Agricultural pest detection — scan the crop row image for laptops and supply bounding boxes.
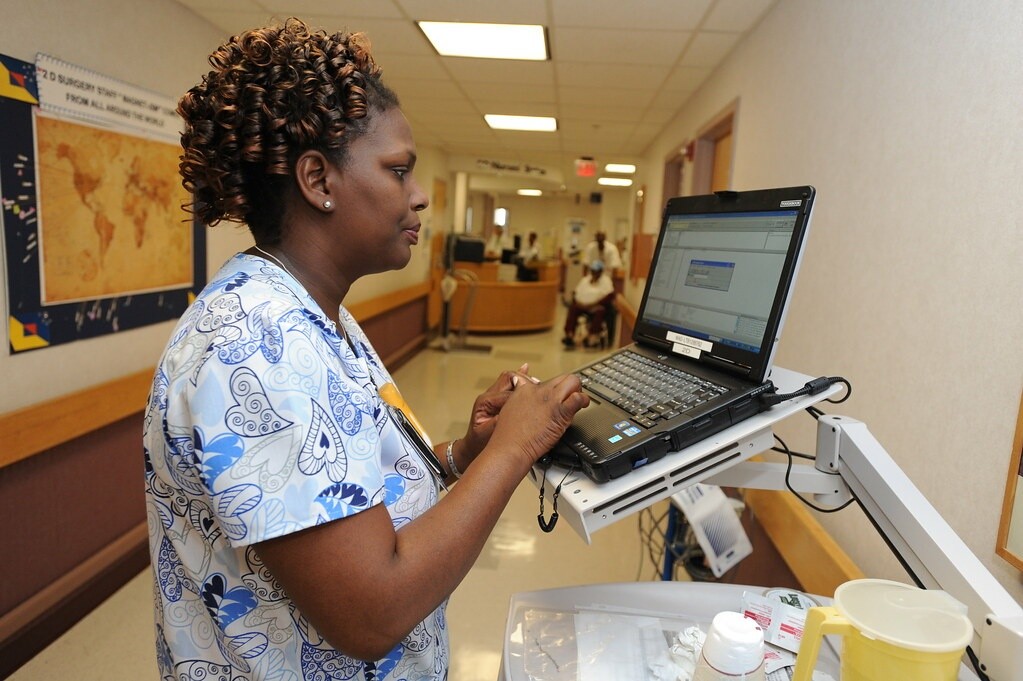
[544,185,816,484]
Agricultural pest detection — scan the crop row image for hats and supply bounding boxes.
[590,260,603,270]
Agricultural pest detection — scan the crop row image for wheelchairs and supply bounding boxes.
[561,294,618,354]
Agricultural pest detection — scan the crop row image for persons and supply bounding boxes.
[484,221,544,283]
[560,260,614,347]
[138,22,594,681]
[580,228,623,347]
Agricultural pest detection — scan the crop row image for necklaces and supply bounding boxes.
[252,245,349,342]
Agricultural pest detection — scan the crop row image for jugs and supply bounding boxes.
[791,578,972,681]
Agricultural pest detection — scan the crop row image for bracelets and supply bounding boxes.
[447,438,467,478]
